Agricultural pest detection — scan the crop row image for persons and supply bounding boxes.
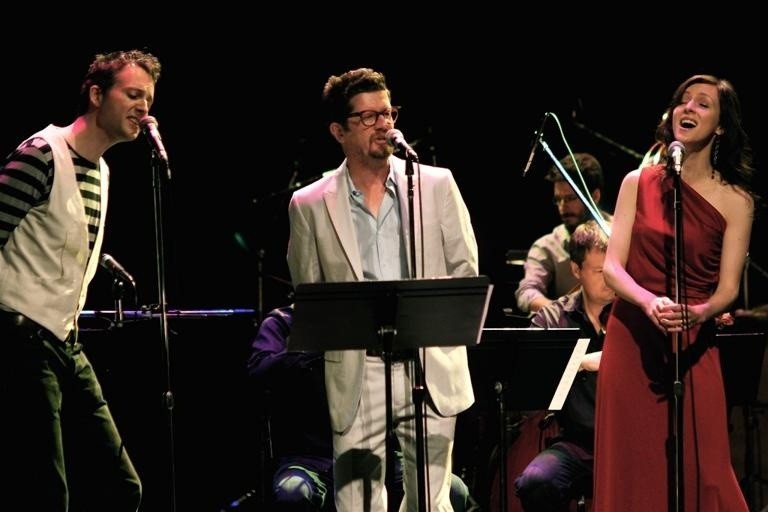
[595,72,761,510]
[240,298,340,510]
[286,66,484,510]
[0,48,162,512]
[512,151,625,333]
[511,220,622,511]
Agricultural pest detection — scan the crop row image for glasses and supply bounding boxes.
[340,105,400,129]
[552,195,580,206]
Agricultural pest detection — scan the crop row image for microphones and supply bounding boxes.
[98,253,134,283]
[427,126,437,166]
[139,116,169,169]
[521,113,550,179]
[385,128,419,161]
[668,141,685,177]
